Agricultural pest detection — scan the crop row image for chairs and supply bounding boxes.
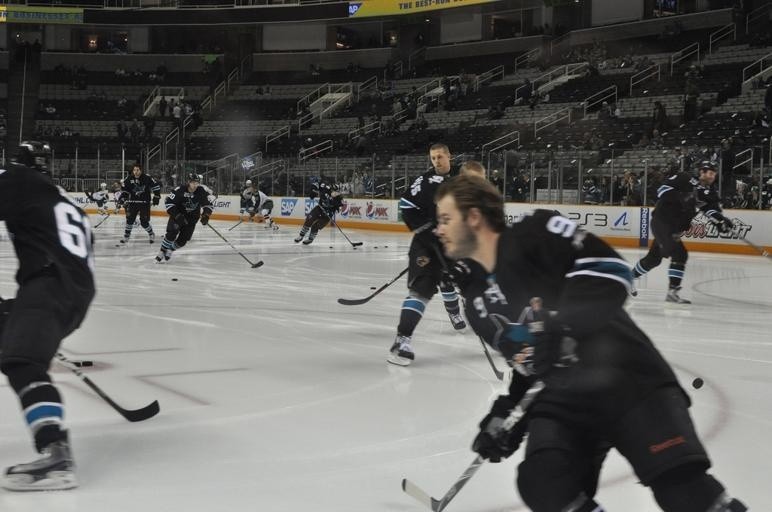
[1,18,771,213]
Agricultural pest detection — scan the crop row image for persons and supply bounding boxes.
[0,164,97,478]
[387,160,487,359]
[432,172,749,511]
[426,143,467,329]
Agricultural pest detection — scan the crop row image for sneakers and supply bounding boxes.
[665,287,691,304]
[155,250,165,262]
[116,239,128,246]
[302,238,313,244]
[391,325,415,361]
[6,440,74,474]
[294,235,303,242]
[448,313,467,330]
[149,232,155,244]
[165,248,173,260]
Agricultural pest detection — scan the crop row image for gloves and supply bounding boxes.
[710,213,733,233]
[471,395,528,463]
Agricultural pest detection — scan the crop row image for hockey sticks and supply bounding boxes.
[399,379,547,512]
[197,213,264,269]
[338,267,408,305]
[316,200,362,247]
[52,350,159,422]
[84,189,146,204]
[431,246,510,382]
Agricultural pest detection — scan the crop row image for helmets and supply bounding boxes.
[329,183,340,196]
[186,172,200,188]
[698,160,719,172]
[10,140,53,179]
